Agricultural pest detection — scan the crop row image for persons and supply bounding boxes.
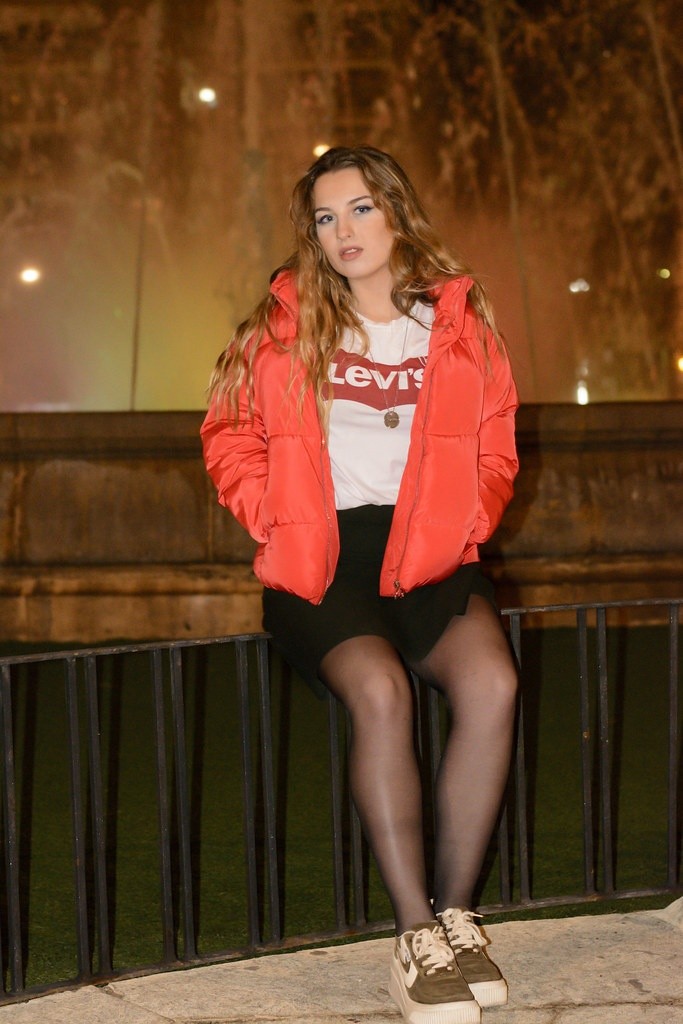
[196,144,523,1024]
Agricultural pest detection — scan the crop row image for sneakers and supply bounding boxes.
[435,905,509,1009]
[388,919,481,1023]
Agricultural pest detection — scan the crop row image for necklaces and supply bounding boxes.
[341,285,419,428]
